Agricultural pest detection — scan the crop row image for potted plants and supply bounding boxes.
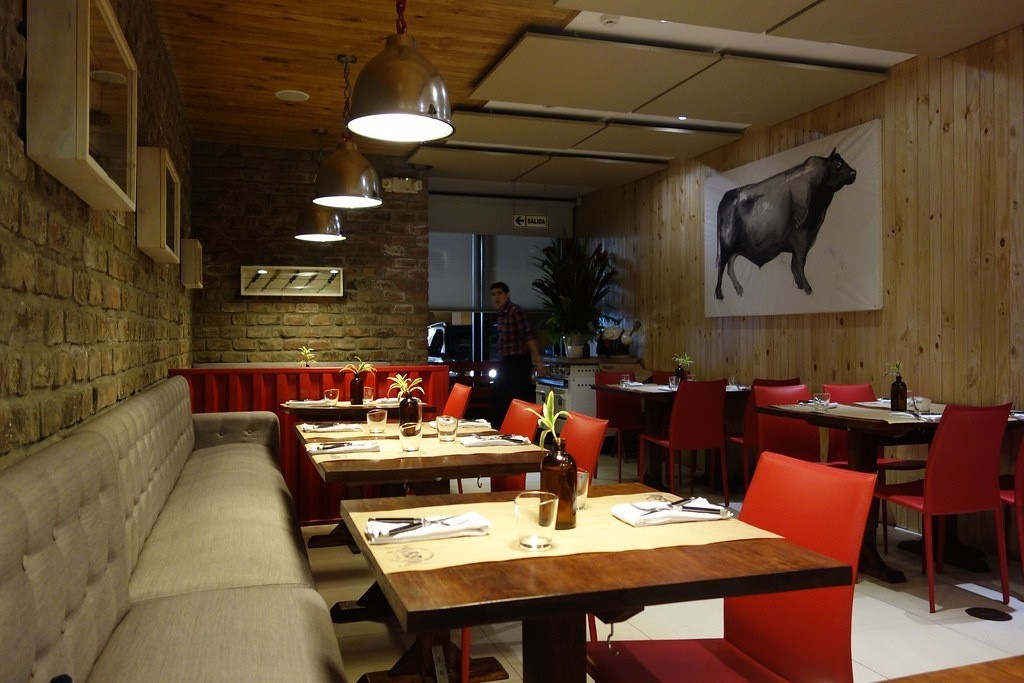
[526,222,618,357]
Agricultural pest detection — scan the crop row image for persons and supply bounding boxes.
[485,282,547,421]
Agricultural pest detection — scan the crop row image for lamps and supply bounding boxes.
[292,0,455,244]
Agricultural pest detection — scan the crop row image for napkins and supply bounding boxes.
[877,397,914,405]
[375,397,421,403]
[458,434,532,446]
[301,423,363,433]
[430,419,491,429]
[611,497,734,527]
[800,399,838,409]
[889,412,943,421]
[658,386,678,391]
[364,509,490,545]
[620,381,643,386]
[286,399,326,405]
[306,440,381,454]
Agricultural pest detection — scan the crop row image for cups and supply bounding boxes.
[812,393,831,414]
[912,393,932,416]
[436,414,458,442]
[513,489,560,550]
[573,466,589,510]
[323,388,339,407]
[366,409,388,432]
[364,386,373,403]
[618,374,630,387]
[687,374,697,381]
[668,375,680,388]
[399,422,421,452]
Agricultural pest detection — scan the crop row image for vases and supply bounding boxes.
[890,376,907,411]
[349,372,363,404]
[398,392,423,431]
[674,364,684,381]
[538,438,577,530]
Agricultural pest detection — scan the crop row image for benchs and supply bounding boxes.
[63,374,317,602]
[2,429,347,683]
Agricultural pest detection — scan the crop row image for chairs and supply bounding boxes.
[427,367,1024,683]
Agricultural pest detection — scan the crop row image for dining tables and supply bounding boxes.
[291,417,551,554]
[338,479,853,683]
[277,395,437,549]
[589,379,753,492]
[754,396,1024,585]
